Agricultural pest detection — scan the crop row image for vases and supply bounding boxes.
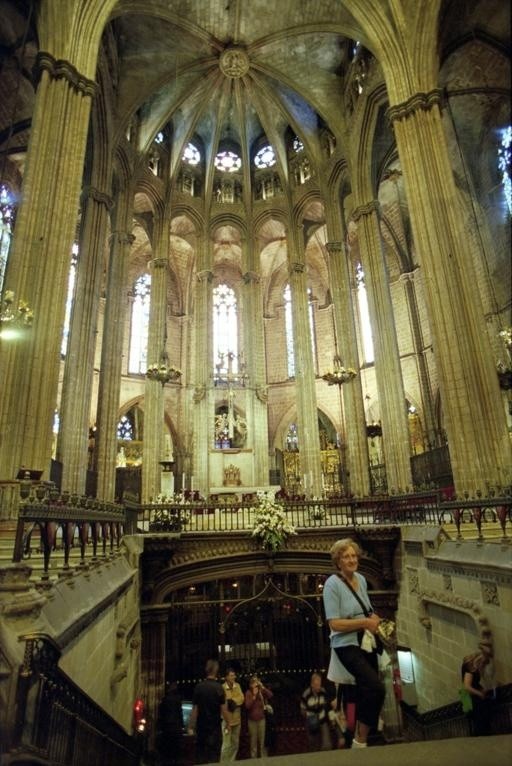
[149,521,181,532]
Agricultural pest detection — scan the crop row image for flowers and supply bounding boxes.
[307,507,327,526]
[146,364,182,382]
[322,368,357,383]
[252,491,298,551]
[153,494,190,523]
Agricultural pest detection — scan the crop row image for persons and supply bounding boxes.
[459,649,493,738]
[322,536,387,748]
[158,659,387,766]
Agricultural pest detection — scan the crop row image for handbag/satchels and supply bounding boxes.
[263,704,274,716]
[308,714,320,732]
[357,629,384,655]
